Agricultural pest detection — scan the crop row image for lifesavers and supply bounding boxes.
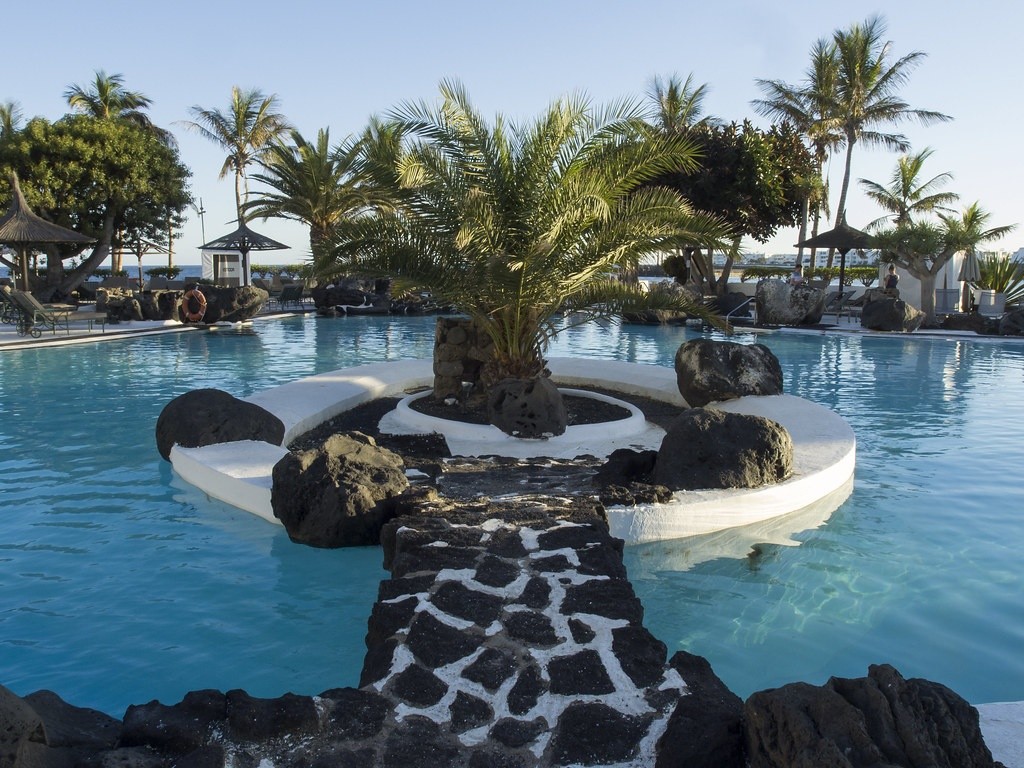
[182,289,207,321]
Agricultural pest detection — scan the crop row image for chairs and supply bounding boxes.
[823,288,864,326]
[250,274,314,309]
[9,289,106,336]
[0,284,77,336]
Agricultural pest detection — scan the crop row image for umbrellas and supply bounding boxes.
[960,237,981,315]
[790,207,895,310]
[0,204,100,338]
[195,218,291,286]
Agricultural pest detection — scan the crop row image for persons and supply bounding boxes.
[790,264,803,285]
[884,264,900,289]
